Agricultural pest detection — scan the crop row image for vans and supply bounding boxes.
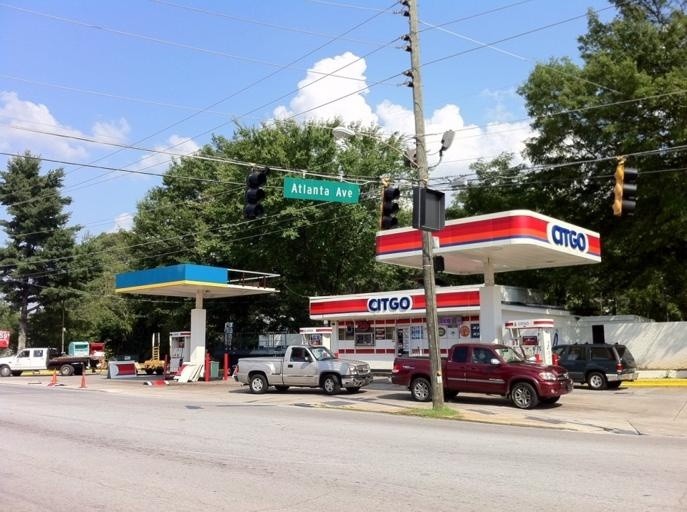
[550,342,637,388]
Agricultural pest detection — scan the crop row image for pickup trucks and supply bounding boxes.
[234,344,373,393]
[390,343,573,409]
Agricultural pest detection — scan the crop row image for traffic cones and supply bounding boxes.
[48,372,56,387]
[80,371,86,389]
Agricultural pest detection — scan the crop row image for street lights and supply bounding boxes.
[331,128,454,408]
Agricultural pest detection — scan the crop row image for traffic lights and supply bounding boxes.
[612,166,641,217]
[379,185,401,234]
[244,171,266,220]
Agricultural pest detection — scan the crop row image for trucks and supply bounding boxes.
[0,348,104,377]
